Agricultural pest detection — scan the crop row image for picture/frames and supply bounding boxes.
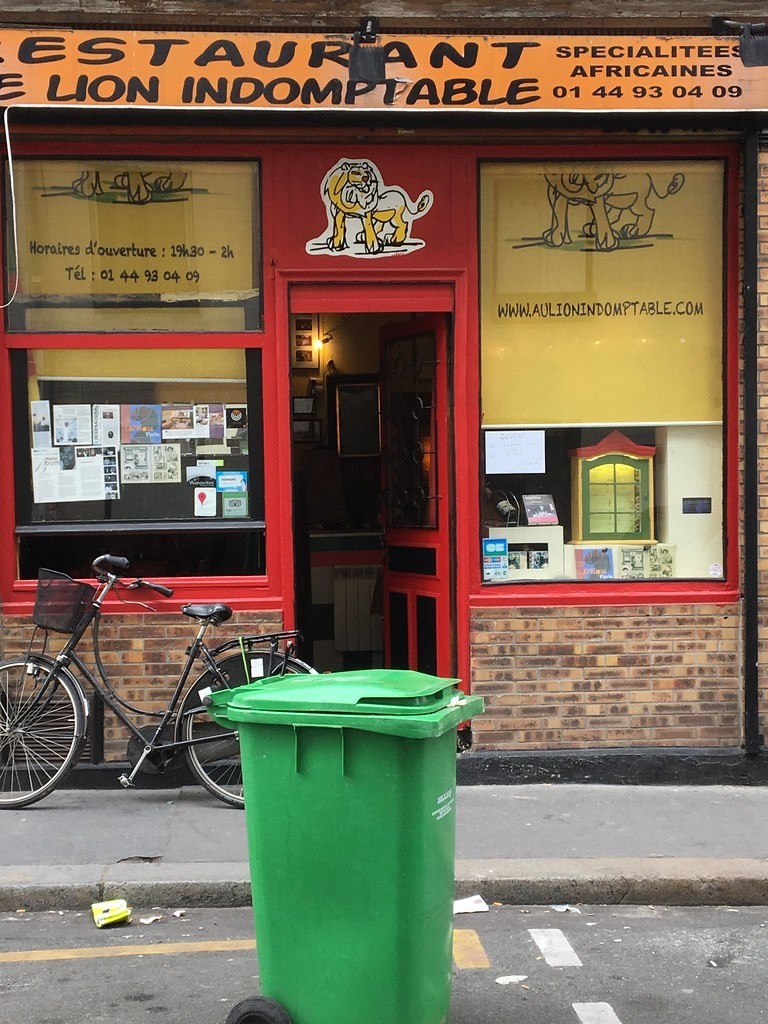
[288,313,320,371]
[293,418,324,449]
[292,396,317,415]
[325,372,389,466]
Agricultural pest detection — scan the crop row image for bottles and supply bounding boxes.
[484,487,517,522]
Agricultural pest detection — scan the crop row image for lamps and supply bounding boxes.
[711,16,768,67]
[348,15,386,85]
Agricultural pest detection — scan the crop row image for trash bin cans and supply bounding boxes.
[203,667,485,1024]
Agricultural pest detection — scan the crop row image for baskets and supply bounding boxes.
[33,567,97,634]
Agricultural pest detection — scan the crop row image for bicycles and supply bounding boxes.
[0,552,321,810]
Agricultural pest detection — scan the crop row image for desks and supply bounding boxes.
[308,523,386,675]
